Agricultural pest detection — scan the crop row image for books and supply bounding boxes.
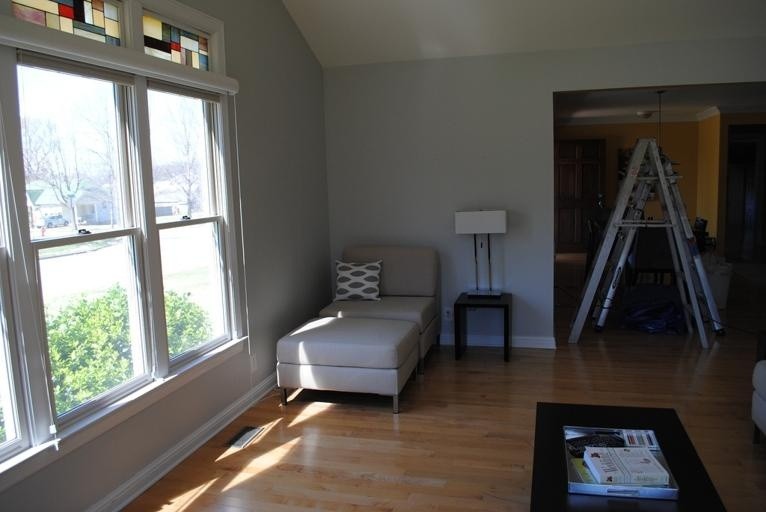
[583,445,670,487]
[563,426,680,500]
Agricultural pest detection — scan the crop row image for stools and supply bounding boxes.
[275,316,419,416]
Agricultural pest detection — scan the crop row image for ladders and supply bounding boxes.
[568,137,710,352]
[593,148,728,338]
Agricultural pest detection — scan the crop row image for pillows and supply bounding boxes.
[331,258,382,301]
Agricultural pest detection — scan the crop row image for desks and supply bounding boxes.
[454,292,513,362]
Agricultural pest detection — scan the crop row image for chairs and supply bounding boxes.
[319,246,441,374]
[586,218,668,296]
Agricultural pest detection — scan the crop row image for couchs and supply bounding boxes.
[748,331,765,444]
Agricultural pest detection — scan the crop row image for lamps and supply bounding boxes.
[636,112,652,118]
[454,209,507,298]
